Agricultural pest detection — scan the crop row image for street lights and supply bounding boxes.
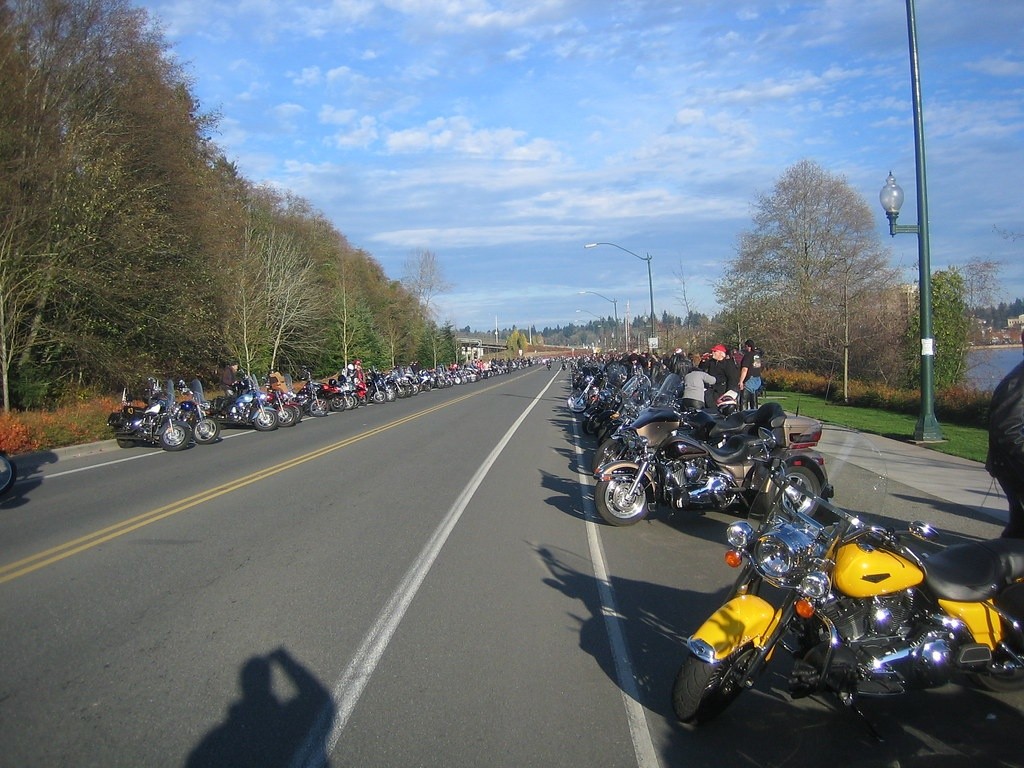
[583,292,618,350]
[880,173,944,441]
[584,243,654,354]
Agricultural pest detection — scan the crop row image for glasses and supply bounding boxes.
[711,350,717,352]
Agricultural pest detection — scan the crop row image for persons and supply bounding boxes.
[985,329,1024,541]
[300,365,311,381]
[347,359,361,383]
[408,356,542,380]
[547,338,765,412]
[221,362,239,396]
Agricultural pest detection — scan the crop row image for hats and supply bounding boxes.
[673,348,682,354]
[231,361,238,366]
[710,344,727,352]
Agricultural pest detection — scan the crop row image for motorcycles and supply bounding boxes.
[0,451,19,493]
[672,430,1024,745]
[108,380,221,452]
[204,353,542,426]
[567,358,836,520]
[547,361,566,370]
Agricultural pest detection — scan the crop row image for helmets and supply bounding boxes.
[179,401,192,411]
[715,395,738,407]
[328,378,337,385]
